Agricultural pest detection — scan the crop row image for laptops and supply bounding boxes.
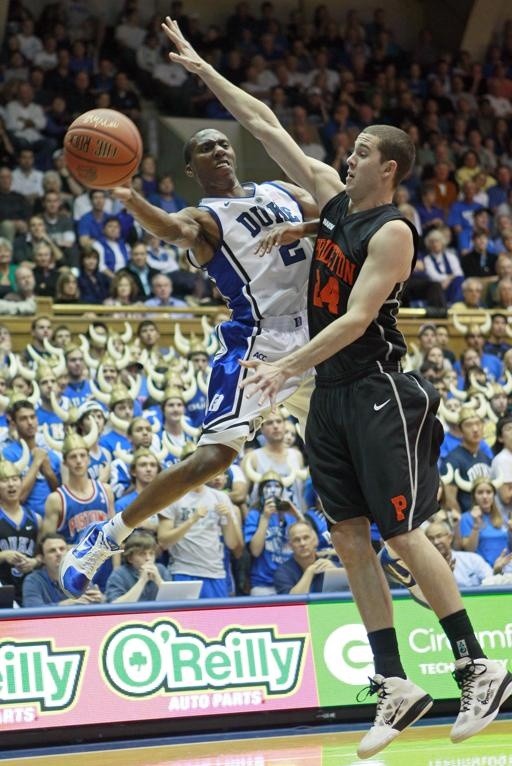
[155,581,203,600]
[322,568,350,592]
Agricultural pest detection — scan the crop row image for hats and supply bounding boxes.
[0,317,218,487]
[409,308,512,492]
[243,456,298,496]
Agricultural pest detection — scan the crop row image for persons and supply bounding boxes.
[60,126,431,609]
[159,12,512,759]
[169,1,512,317]
[0,311,342,608]
[2,3,229,303]
[400,311,512,585]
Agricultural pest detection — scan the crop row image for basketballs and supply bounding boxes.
[65,109,143,186]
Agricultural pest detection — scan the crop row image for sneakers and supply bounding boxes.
[355,672,435,761]
[57,519,122,601]
[376,543,434,611]
[448,654,512,744]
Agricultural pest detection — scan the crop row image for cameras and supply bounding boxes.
[275,498,290,512]
